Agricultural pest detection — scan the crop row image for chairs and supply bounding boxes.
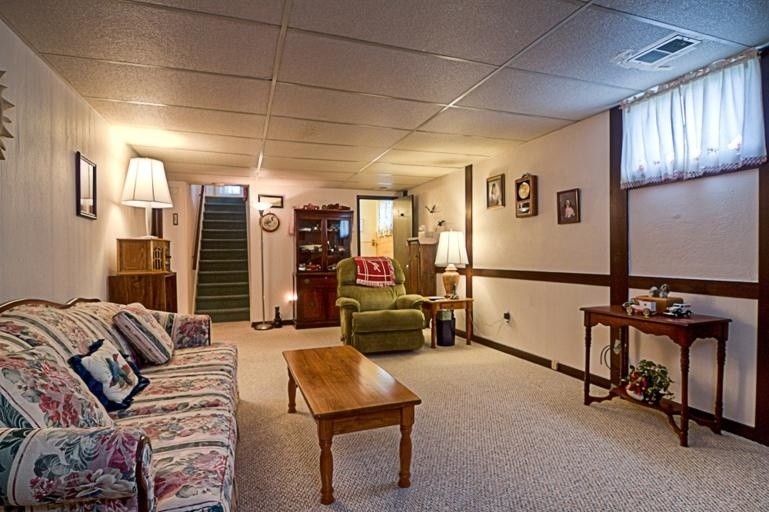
[335,256,429,354]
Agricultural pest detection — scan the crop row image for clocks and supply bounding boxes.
[515,173,538,218]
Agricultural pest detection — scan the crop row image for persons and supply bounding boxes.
[565,199,576,219]
[490,183,500,201]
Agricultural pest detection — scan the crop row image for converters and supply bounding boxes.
[504,313,509,319]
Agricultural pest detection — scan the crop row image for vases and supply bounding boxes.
[625,385,665,405]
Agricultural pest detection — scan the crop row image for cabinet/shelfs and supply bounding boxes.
[108,239,177,313]
[293,209,354,329]
[406,244,437,328]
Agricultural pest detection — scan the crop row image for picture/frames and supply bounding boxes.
[76,151,97,219]
[173,213,178,225]
[258,194,283,208]
[487,173,505,208]
[556,188,580,224]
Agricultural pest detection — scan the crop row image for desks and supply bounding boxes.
[422,296,474,349]
[579,305,733,447]
[282,344,421,505]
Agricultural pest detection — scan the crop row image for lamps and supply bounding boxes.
[434,228,470,298]
[255,202,273,330]
[121,157,174,239]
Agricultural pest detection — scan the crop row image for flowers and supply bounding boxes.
[620,360,676,404]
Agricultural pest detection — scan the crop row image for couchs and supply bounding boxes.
[0,297,240,512]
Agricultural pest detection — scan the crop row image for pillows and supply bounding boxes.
[68,338,150,412]
[0,343,116,429]
[112,301,174,365]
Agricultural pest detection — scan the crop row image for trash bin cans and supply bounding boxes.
[436,316,455,346]
